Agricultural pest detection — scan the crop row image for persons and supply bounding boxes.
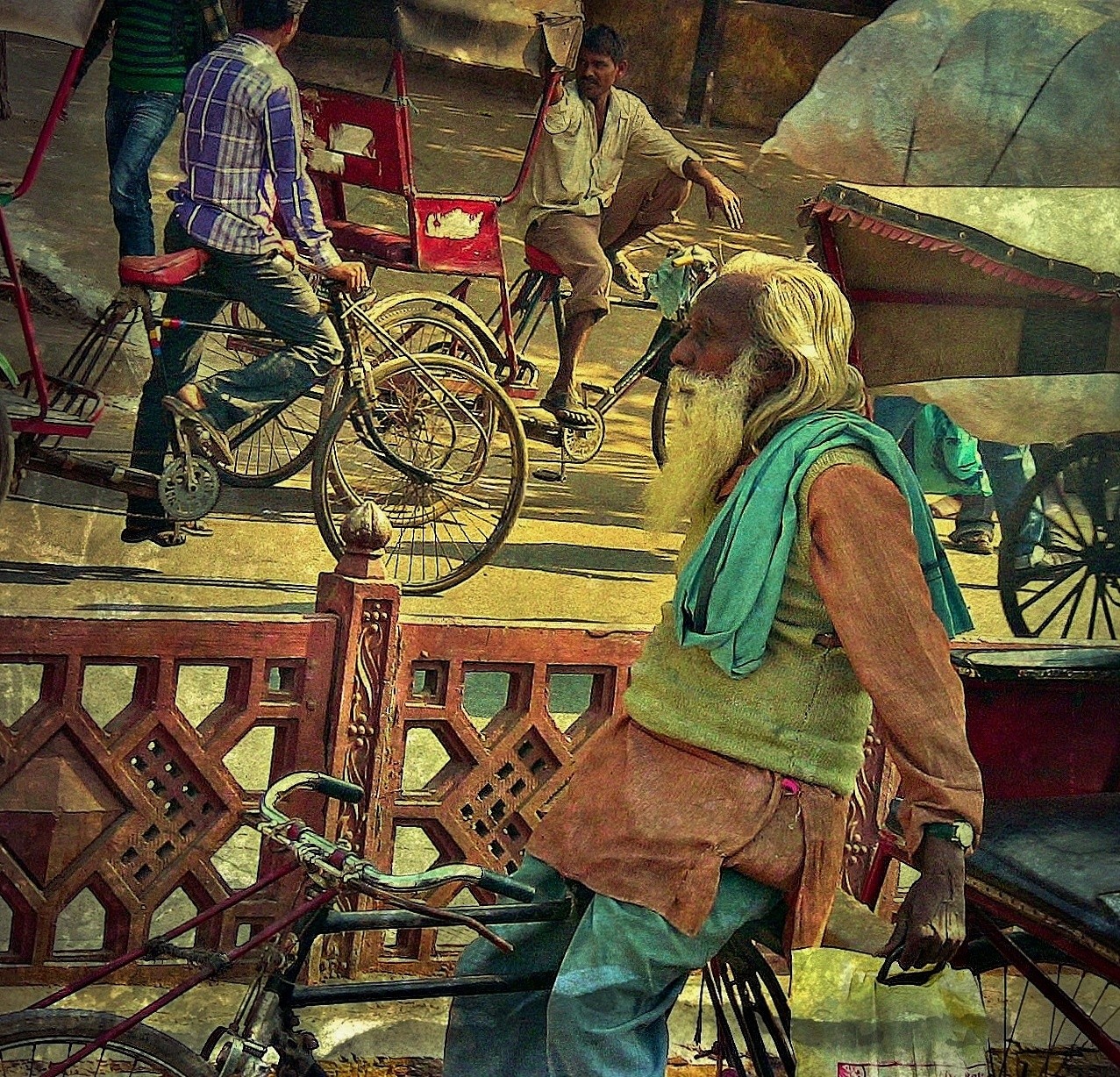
[514,21,744,429]
[118,0,370,549]
[439,244,986,1077]
[930,430,1081,572]
[71,0,231,289]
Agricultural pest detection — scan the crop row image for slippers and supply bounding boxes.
[603,249,643,293]
[539,397,596,431]
[120,517,214,544]
[162,394,235,464]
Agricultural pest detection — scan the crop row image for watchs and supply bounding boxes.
[923,817,975,851]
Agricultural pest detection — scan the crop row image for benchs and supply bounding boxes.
[295,78,521,386]
[859,639,1120,982]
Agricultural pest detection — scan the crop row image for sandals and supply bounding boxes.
[959,533,995,556]
[1040,527,1074,570]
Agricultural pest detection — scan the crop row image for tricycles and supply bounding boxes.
[0,0,528,596]
[181,0,721,487]
[0,187,1120,1076]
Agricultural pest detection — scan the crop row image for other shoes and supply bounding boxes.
[108,291,164,324]
